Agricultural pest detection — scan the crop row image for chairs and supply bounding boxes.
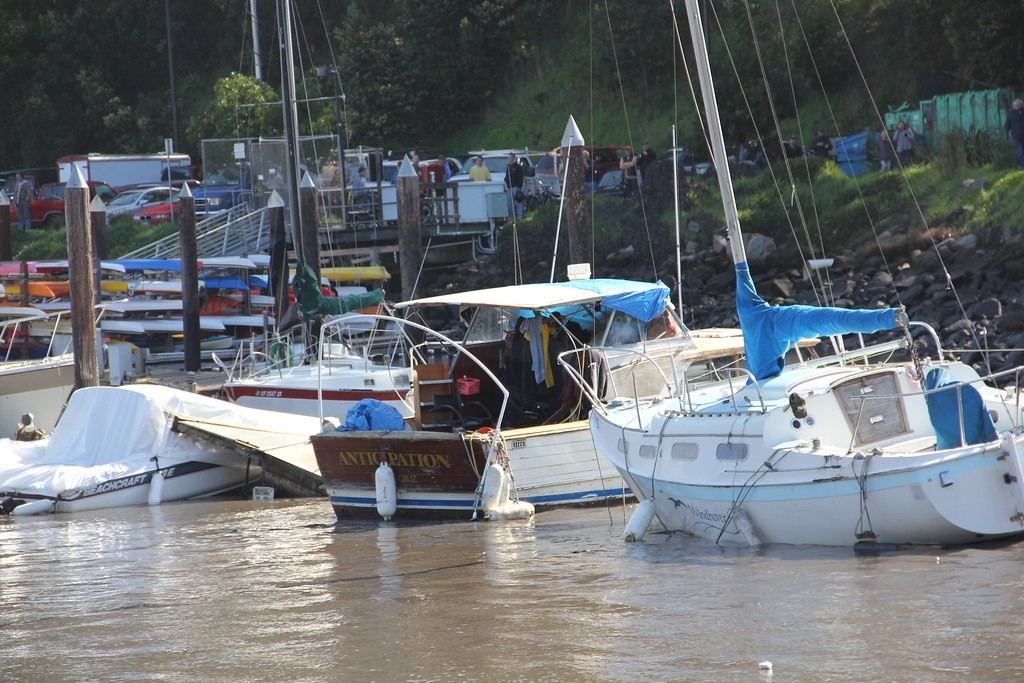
[432,392,493,431]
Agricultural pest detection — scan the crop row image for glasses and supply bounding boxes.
[336,173,340,176]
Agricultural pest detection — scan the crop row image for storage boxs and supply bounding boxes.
[458,378,480,395]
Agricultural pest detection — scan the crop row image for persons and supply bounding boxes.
[504,153,523,219]
[583,151,596,194]
[620,146,637,196]
[637,142,656,183]
[391,161,402,184]
[893,121,914,168]
[812,129,833,157]
[412,154,450,196]
[780,135,803,158]
[469,156,491,181]
[1005,99,1024,167]
[13,173,33,231]
[346,167,379,227]
[877,131,892,172]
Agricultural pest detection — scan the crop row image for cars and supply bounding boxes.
[7,180,117,233]
[300,161,328,205]
[4,174,39,198]
[105,186,180,226]
[378,135,810,197]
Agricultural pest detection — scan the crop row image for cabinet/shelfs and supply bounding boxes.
[413,362,453,430]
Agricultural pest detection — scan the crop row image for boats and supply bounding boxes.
[0,257,391,366]
[0,383,340,515]
[310,258,823,522]
[224,339,415,420]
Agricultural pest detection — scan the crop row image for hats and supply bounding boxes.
[21,413,34,421]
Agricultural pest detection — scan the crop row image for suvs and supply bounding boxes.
[190,162,279,221]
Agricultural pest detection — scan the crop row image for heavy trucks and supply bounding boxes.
[55,148,202,187]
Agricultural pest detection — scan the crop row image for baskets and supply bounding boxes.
[457,377,480,396]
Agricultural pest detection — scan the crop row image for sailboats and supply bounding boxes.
[558,0,1022,552]
